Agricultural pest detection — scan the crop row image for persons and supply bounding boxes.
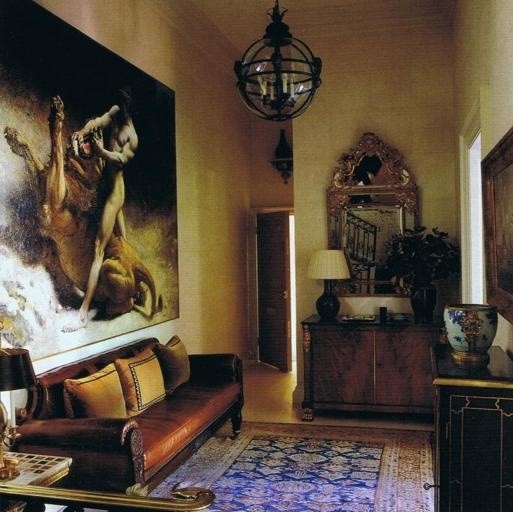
[61,85,139,332]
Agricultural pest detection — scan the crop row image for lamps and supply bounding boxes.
[307,250,351,319]
[0,349,37,482]
[268,129,294,184]
[234,1,323,122]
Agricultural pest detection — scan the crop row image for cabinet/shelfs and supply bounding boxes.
[431,345,512,512]
[300,314,448,421]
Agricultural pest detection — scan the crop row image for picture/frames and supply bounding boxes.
[480,126,513,327]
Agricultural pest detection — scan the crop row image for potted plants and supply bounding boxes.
[386,225,459,321]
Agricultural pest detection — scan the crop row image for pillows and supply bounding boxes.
[114,346,166,418]
[155,335,191,393]
[63,363,127,423]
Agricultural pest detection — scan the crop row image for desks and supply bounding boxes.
[0,451,73,511]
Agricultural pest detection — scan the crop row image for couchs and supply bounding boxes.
[9,339,243,498]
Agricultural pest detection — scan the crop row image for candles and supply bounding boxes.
[10,391,15,427]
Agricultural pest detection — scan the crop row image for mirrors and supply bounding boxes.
[326,132,421,298]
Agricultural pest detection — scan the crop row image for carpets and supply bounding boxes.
[67,418,435,512]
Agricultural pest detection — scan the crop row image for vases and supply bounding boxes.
[441,304,498,363]
